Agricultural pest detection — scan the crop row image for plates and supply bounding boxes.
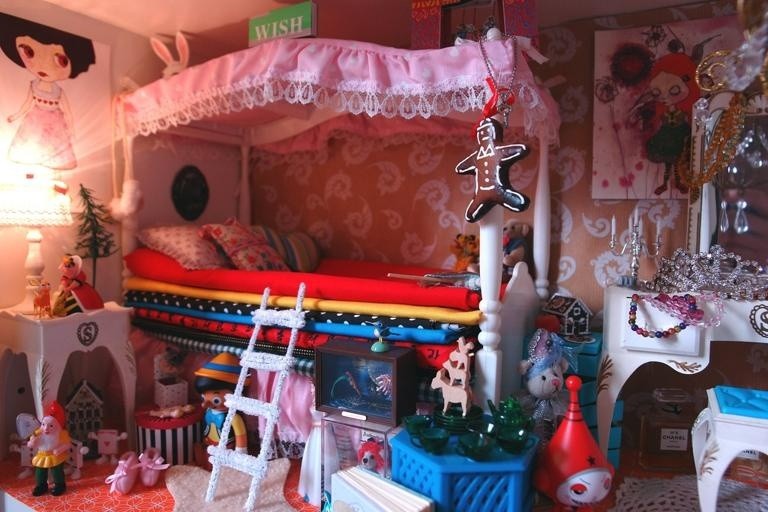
[433,402,485,435]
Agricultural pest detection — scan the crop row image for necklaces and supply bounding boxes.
[679,90,746,190]
[628,290,696,339]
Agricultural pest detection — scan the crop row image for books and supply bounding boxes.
[331,463,436,512]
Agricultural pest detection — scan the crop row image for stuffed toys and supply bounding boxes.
[518,327,574,424]
[356,438,391,478]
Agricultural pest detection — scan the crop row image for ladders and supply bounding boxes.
[203,283,311,512]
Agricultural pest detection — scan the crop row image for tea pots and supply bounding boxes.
[487,394,536,436]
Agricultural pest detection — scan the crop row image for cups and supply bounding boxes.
[457,427,492,463]
[491,428,537,457]
[408,428,449,456]
[400,414,433,436]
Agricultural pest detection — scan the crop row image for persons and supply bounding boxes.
[27,399,74,496]
[192,350,248,473]
[52,252,105,318]
